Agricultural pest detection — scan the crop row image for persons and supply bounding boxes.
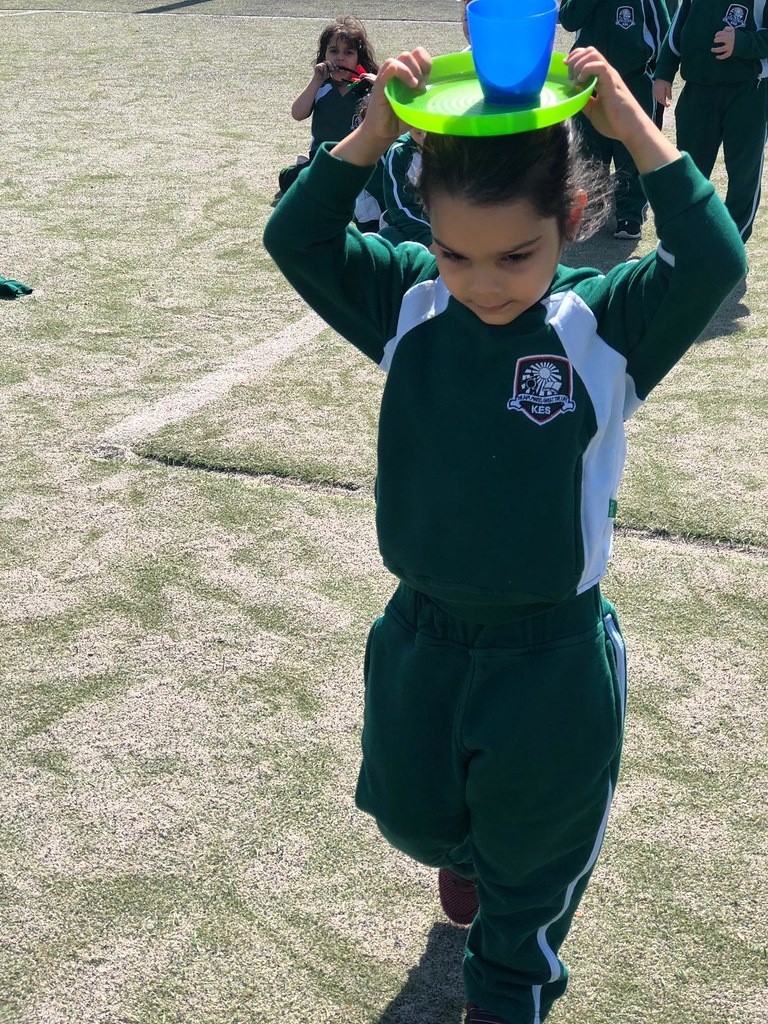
[460,0,471,52]
[559,0,671,240]
[651,0,768,242]
[279,15,432,247]
[263,45,749,1024]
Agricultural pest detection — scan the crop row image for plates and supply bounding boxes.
[384,52,598,137]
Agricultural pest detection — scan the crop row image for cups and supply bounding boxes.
[466,0,559,108]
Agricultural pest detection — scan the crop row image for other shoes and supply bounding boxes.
[438,867,479,924]
[465,1003,508,1024]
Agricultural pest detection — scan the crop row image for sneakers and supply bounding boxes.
[614,220,641,239]
